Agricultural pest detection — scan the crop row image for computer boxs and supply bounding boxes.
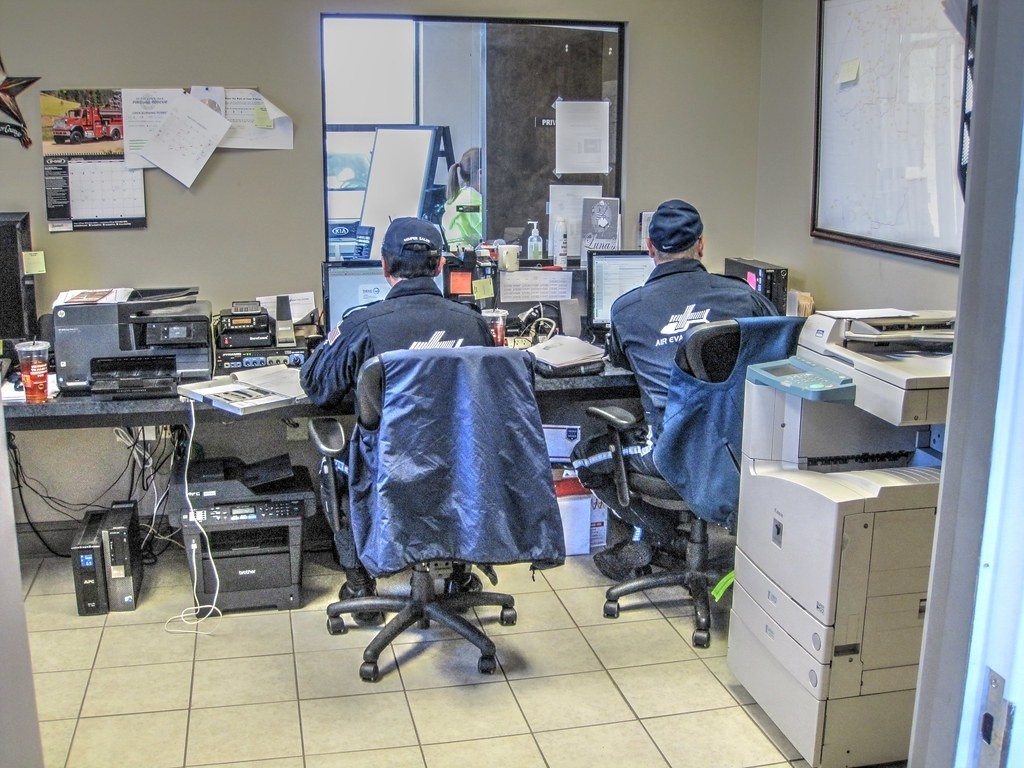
[724,258,788,315]
[0,212,39,368]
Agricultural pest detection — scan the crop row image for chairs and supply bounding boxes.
[326,346,567,682]
[388,214,451,253]
[586,316,808,648]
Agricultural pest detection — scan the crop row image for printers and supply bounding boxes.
[727,310,955,768]
[53,285,212,390]
[168,452,318,620]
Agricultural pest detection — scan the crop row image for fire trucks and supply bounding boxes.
[51,92,123,146]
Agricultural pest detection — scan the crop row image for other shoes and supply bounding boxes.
[650,529,690,560]
[444,572,483,613]
[339,581,380,625]
[593,539,652,582]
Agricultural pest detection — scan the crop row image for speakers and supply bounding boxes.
[559,299,581,338]
[276,296,296,347]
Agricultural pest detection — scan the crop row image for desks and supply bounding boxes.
[3,361,641,431]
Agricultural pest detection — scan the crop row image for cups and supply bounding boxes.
[15,341,51,404]
[481,309,509,347]
[498,245,519,271]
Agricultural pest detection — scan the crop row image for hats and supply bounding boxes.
[648,199,703,253]
[381,217,442,257]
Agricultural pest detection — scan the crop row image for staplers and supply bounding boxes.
[475,249,492,266]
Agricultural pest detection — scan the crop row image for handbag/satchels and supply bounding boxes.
[526,334,605,378]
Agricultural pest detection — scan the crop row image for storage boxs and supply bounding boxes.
[543,425,608,556]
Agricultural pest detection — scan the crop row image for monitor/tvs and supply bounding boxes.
[587,250,657,331]
[321,257,450,336]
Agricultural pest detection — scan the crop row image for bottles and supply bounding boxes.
[554,217,568,270]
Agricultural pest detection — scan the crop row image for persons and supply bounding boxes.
[441,147,484,254]
[300,217,495,628]
[570,200,781,582]
